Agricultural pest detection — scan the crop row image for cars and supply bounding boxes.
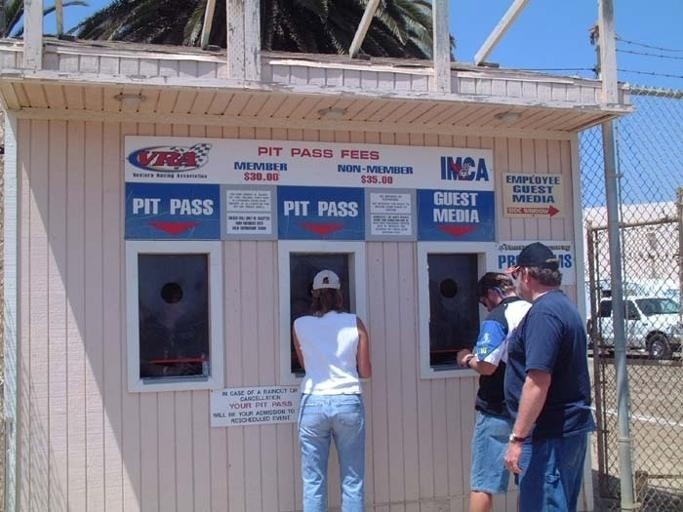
[587,295,683,359]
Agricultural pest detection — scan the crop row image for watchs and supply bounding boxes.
[508,433,526,443]
[466,356,474,368]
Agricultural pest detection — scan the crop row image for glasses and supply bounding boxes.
[511,269,520,279]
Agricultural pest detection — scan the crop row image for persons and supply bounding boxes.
[292,270,371,512]
[456,272,533,512]
[504,242,595,512]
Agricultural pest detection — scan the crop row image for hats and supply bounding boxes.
[313,270,340,290]
[517,243,556,267]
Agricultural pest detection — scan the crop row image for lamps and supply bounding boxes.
[494,111,521,122]
[317,107,349,121]
[113,94,144,109]
